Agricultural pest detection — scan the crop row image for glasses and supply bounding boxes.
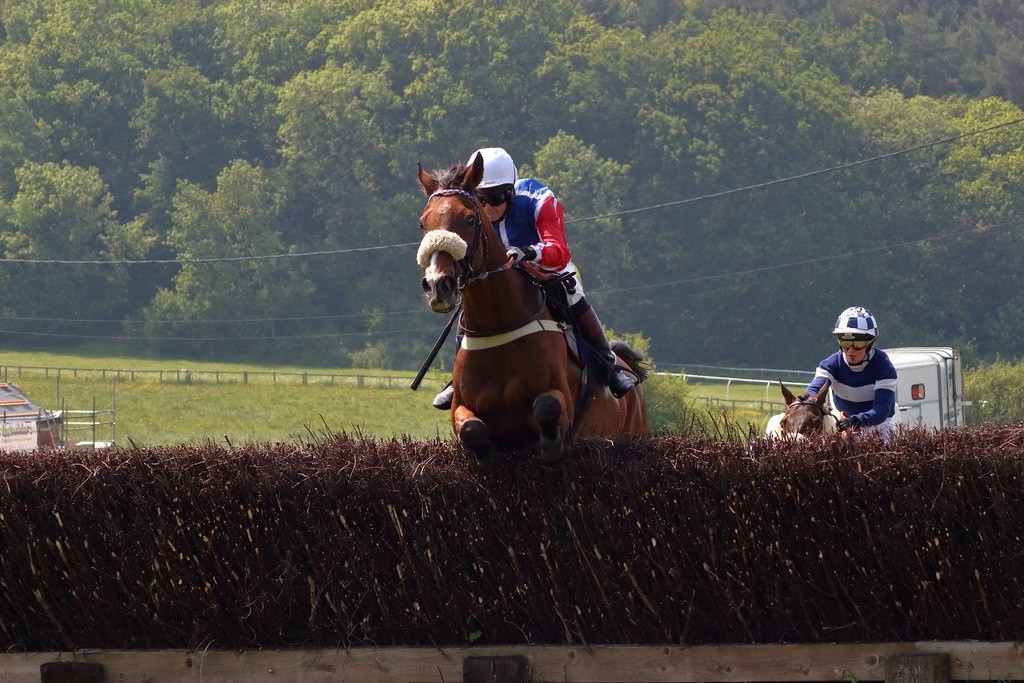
[837,339,868,350]
[472,188,509,207]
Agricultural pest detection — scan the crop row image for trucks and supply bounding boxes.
[883,346,965,434]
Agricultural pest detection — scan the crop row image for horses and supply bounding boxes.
[415,151,650,439]
[779,377,853,441]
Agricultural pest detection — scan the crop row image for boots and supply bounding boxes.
[575,306,634,399]
[432,386,456,410]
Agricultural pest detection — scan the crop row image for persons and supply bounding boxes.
[802,305,900,448]
[432,147,636,410]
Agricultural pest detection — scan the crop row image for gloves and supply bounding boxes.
[506,246,536,266]
[837,417,859,431]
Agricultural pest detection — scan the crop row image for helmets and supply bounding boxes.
[466,147,518,188]
[832,306,880,341]
